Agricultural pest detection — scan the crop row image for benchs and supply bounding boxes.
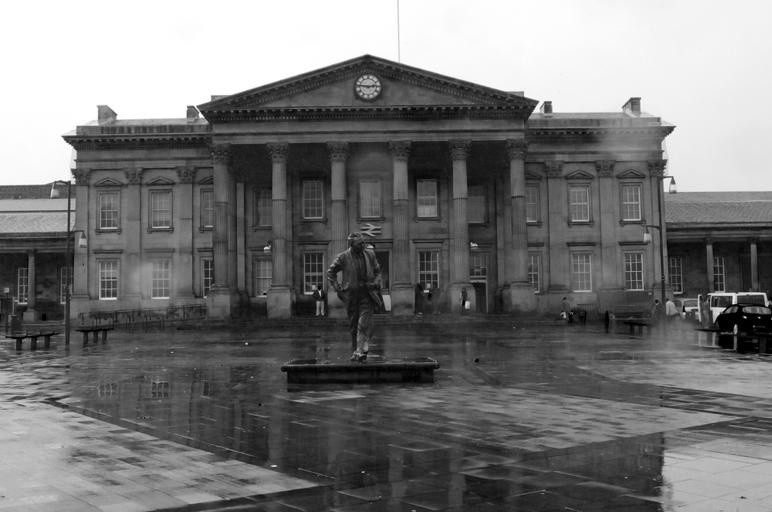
[622,317,658,337]
[75,324,114,347]
[5,327,61,353]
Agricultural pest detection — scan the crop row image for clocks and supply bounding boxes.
[352,70,384,103]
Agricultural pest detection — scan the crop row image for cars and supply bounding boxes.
[674,290,772,340]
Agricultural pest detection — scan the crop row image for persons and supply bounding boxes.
[415,282,426,315]
[665,298,678,319]
[312,285,326,317]
[556,295,617,335]
[651,299,660,317]
[326,232,383,360]
[431,283,440,315]
[459,287,468,312]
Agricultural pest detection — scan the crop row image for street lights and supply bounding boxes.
[50,178,87,347]
[644,174,679,322]
